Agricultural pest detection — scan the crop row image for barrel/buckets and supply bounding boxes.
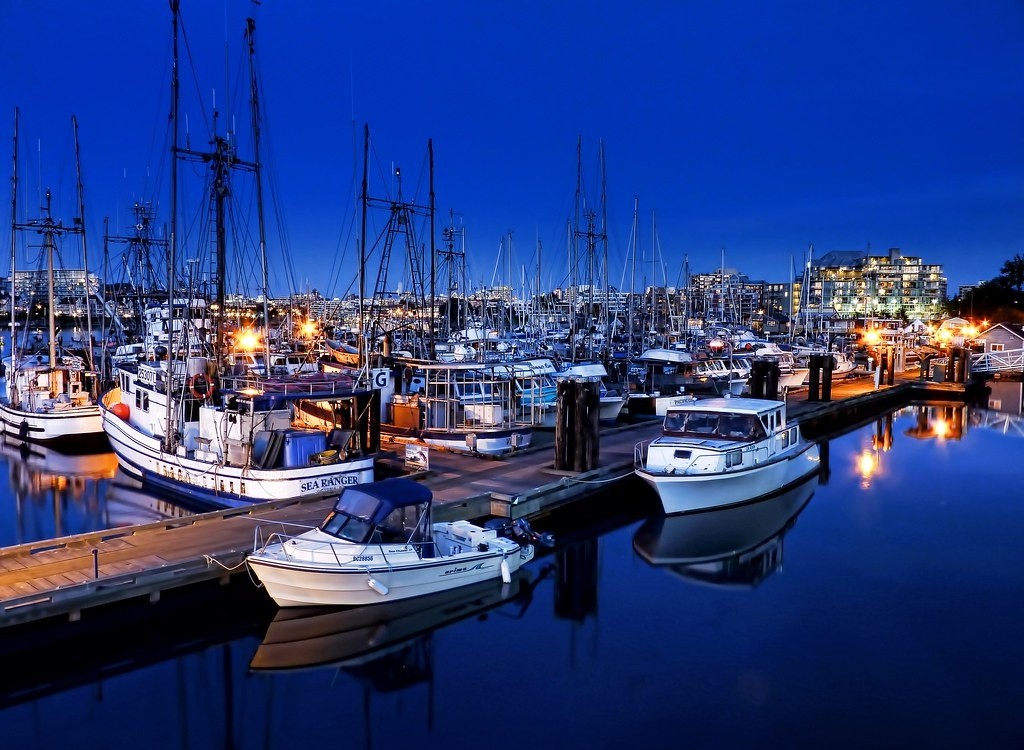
[320,450,338,465]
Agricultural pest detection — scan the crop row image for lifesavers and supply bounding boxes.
[190,373,215,398]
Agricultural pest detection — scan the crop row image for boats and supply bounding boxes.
[1,0,1024,674]
